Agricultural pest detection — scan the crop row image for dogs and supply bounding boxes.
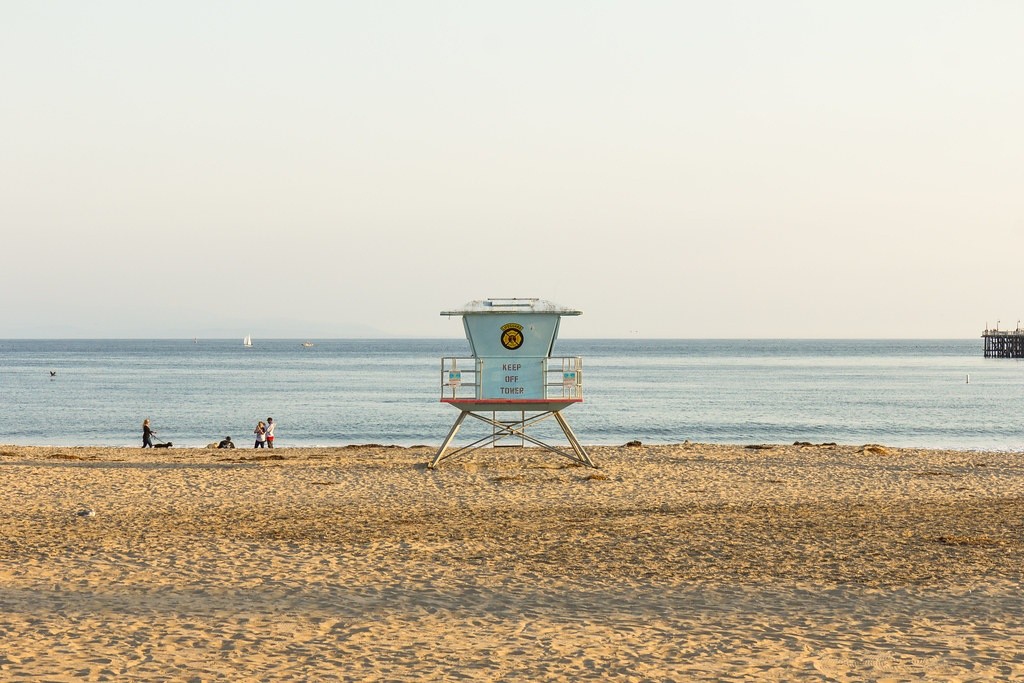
[151,442,173,449]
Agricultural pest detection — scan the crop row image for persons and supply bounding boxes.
[142,418,157,448]
[265,417,273,448]
[254,421,265,448]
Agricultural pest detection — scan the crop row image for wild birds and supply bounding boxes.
[50,371,56,377]
